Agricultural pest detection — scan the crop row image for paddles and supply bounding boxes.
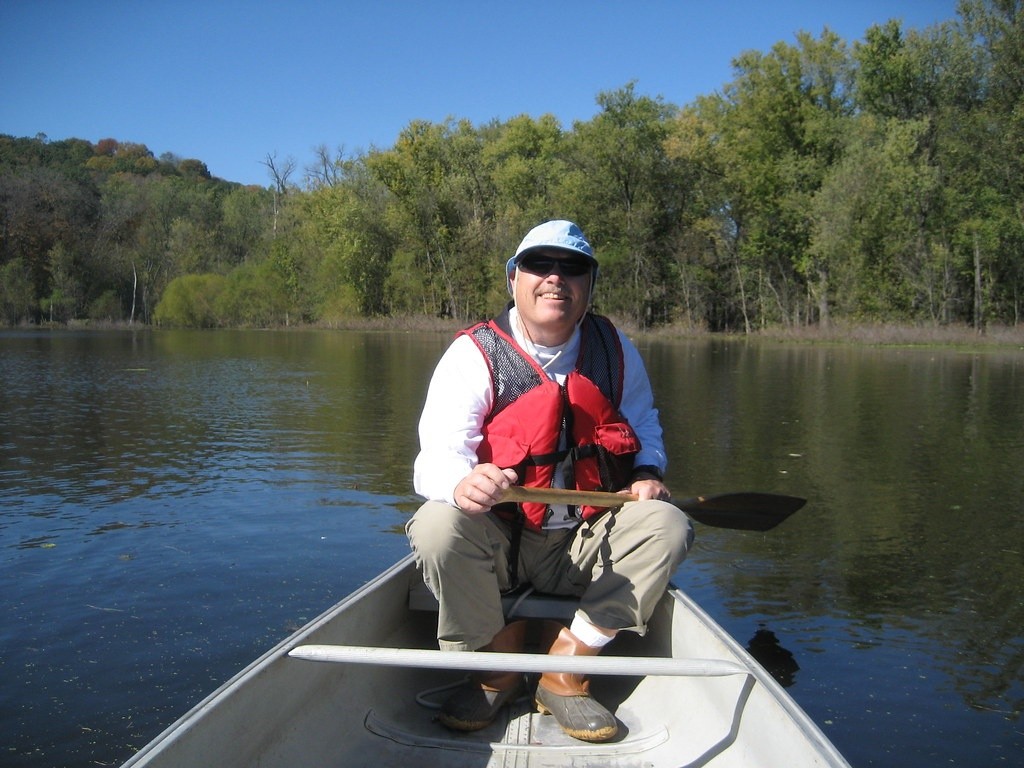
[492,486,808,532]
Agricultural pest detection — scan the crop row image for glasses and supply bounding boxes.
[519,253,589,276]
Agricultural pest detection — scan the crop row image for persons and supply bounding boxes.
[404,220,697,741]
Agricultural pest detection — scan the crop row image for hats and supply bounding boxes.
[506,220,598,300]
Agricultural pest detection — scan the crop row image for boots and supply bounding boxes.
[438,617,528,730]
[529,619,618,741]
